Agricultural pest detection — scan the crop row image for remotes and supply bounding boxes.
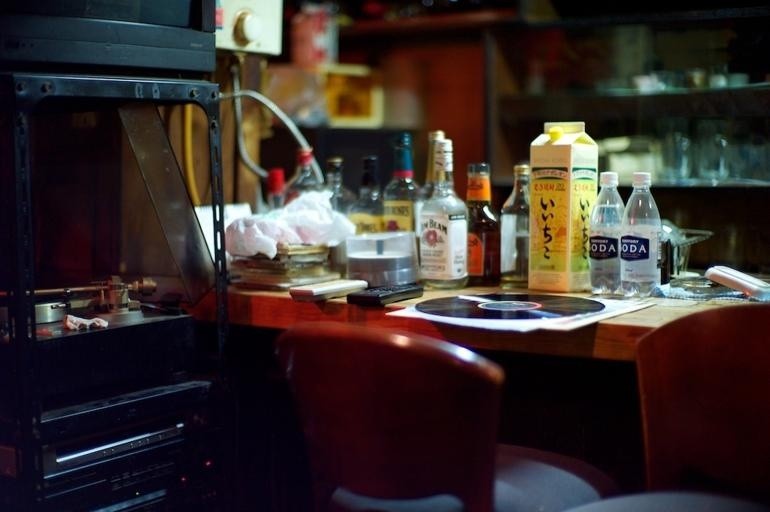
[289,278,368,298]
[348,282,424,303]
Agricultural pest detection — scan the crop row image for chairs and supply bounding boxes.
[573,301,769,511]
[275,319,597,511]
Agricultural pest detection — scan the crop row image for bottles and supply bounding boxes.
[588,170,627,297]
[289,2,343,65]
[619,175,663,298]
[264,128,529,292]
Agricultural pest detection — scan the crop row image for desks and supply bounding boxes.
[184,277,750,361]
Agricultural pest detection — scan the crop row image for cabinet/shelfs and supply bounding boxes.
[324,0,770,282]
[3,75,232,512]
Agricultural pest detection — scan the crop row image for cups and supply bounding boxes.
[659,116,770,181]
